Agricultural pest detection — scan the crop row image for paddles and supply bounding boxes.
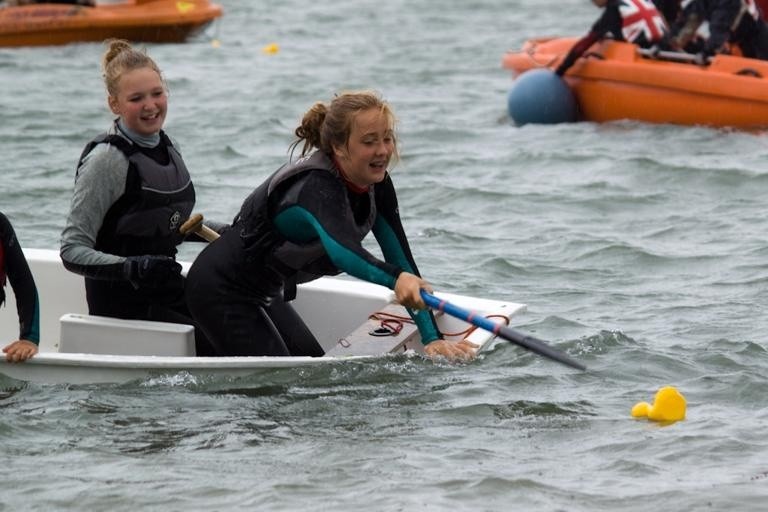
[420,287,587,371]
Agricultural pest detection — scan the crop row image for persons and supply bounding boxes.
[647,0,768,67]
[59,39,233,358]
[183,91,484,369]
[551,0,680,79]
[0,211,41,365]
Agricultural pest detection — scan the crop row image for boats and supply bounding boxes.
[0,0,223,46]
[0,243,526,383]
[500,31,767,132]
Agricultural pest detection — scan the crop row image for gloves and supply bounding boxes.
[123,255,182,301]
